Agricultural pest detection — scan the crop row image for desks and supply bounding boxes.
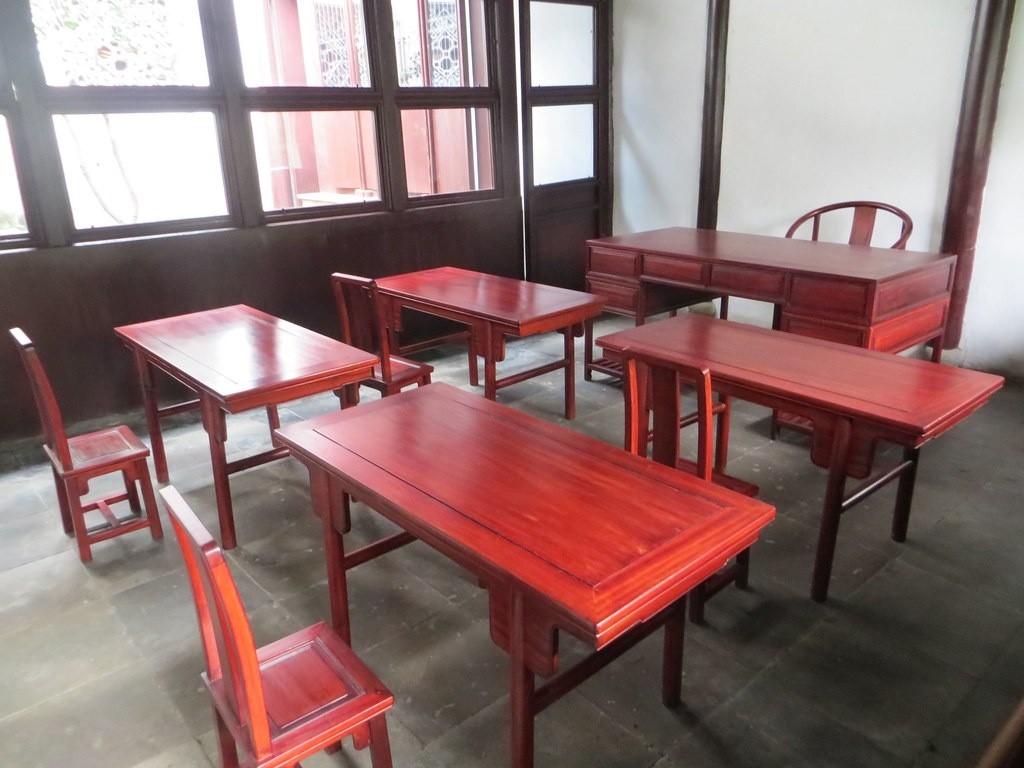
[113,225,1004,768]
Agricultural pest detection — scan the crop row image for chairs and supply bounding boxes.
[158,484,394,768]
[7,326,164,563]
[330,272,434,397]
[622,345,759,624]
[785,200,913,249]
